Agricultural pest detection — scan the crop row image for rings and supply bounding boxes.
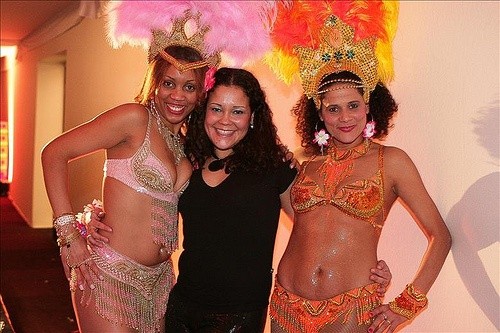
[86,234,92,239]
[71,262,79,270]
[382,313,388,320]
[385,319,391,325]
[77,259,86,268]
[85,258,93,263]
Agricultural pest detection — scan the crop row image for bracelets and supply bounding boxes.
[53,214,76,230]
[55,230,81,247]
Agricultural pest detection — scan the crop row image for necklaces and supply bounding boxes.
[208,150,235,171]
[150,99,187,165]
[317,139,374,202]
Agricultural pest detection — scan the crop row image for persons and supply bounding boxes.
[268,38,451,333]
[40,44,301,333]
[75,66,393,333]
